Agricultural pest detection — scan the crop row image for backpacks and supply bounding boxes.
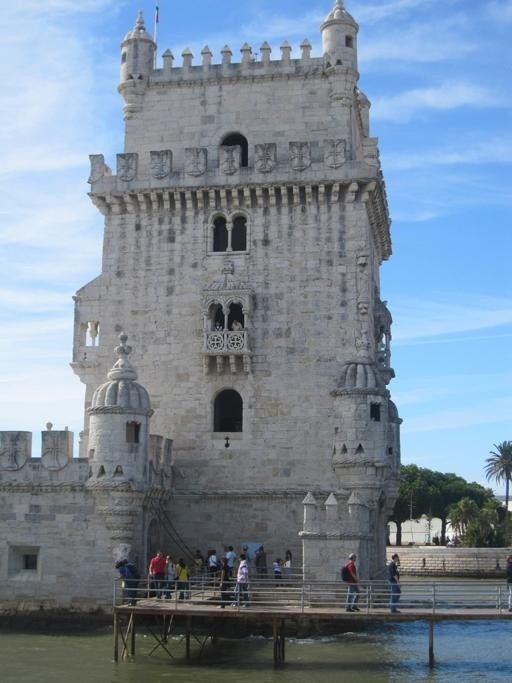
[341,564,352,581]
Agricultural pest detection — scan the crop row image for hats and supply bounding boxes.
[349,553,355,559]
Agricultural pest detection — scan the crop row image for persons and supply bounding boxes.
[387,550,403,613]
[213,319,223,330]
[341,551,364,611]
[230,317,244,330]
[504,553,511,612]
[113,541,293,609]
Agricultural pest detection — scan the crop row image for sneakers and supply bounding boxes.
[128,603,136,606]
[509,608,512,612]
[230,604,236,607]
[179,595,189,600]
[346,608,354,612]
[243,605,250,608]
[352,606,360,611]
[157,595,172,600]
[217,606,225,609]
[391,610,400,614]
